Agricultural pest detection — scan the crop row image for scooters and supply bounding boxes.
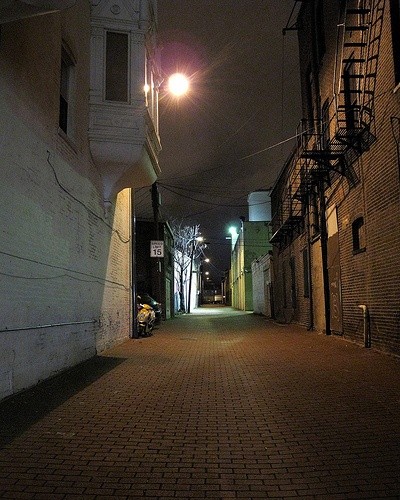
[137,303,156,337]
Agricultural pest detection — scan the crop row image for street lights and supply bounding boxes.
[185,236,205,313]
